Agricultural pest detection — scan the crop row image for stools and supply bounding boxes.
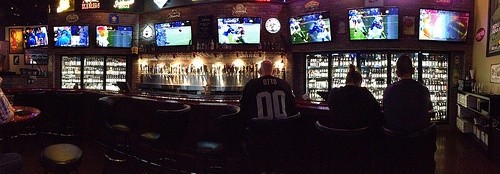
[39,143,86,174]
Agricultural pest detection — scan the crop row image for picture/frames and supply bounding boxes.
[486,0,500,57]
[490,63,500,84]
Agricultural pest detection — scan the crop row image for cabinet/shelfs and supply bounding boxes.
[456,91,500,155]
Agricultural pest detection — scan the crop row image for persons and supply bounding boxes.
[289,15,331,43]
[0,77,14,124]
[328,65,382,129]
[383,55,435,132]
[24,27,47,47]
[238,60,296,127]
[54,26,71,47]
[223,25,245,43]
[349,8,385,39]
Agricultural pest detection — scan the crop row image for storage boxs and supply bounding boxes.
[456,117,473,133]
[457,93,477,108]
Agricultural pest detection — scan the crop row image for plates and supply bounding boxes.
[8,106,41,122]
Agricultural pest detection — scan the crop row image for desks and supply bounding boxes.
[0,105,40,153]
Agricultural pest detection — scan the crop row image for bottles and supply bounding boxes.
[62,57,126,93]
[306,54,448,122]
[452,56,460,88]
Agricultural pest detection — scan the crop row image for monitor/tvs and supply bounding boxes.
[418,8,470,44]
[23,25,49,48]
[348,6,400,41]
[288,11,333,45]
[216,16,262,45]
[154,19,194,48]
[95,25,133,49]
[52,25,90,48]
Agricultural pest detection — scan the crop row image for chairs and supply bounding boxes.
[96,96,133,157]
[247,111,301,161]
[192,102,241,174]
[382,122,437,174]
[315,121,370,174]
[138,99,191,166]
